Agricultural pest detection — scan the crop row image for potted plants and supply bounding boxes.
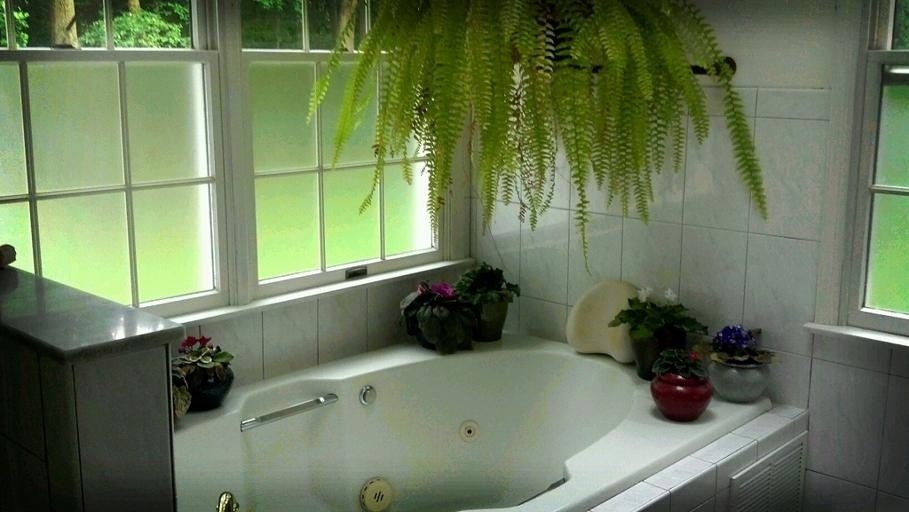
[454,262,520,341]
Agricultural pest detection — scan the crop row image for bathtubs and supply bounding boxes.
[172,331,773,512]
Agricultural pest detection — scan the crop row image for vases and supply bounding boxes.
[407,307,469,353]
[651,373,713,422]
[629,326,686,383]
[184,367,234,413]
[707,362,770,402]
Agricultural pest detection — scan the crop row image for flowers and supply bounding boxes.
[608,287,708,341]
[171,335,234,376]
[692,324,783,365]
[400,280,455,303]
[651,349,710,384]
